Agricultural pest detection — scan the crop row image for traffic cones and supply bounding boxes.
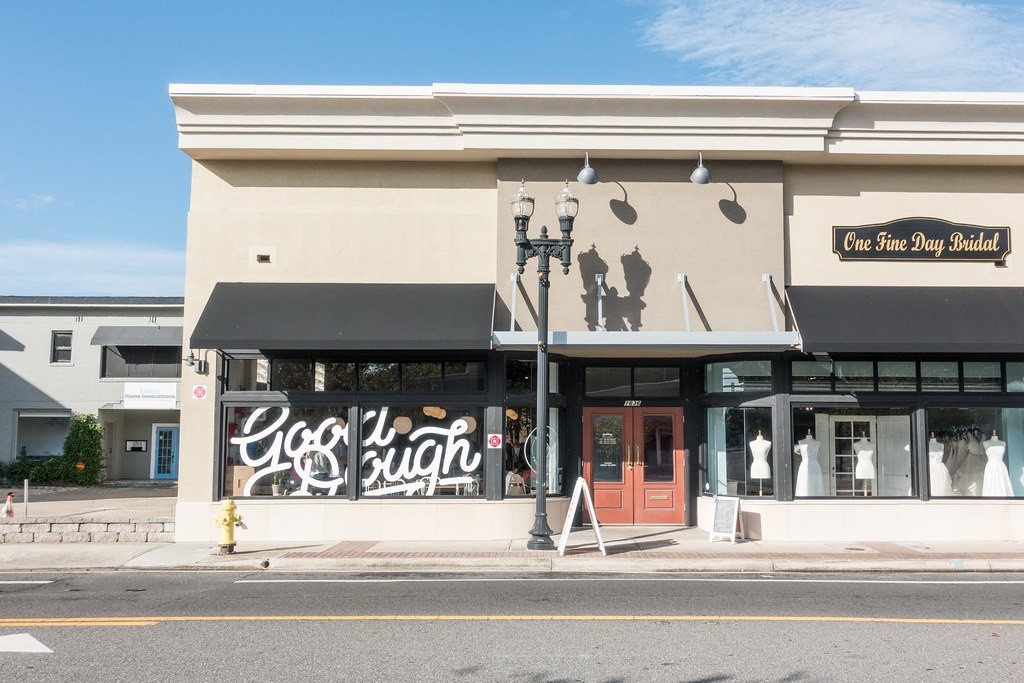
[2,495,15,518]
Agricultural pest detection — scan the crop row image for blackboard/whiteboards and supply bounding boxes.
[559,475,607,557]
[710,494,744,538]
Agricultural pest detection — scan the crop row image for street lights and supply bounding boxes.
[509,177,580,550]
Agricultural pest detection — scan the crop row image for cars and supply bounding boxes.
[285,462,392,496]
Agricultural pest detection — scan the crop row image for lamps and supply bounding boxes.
[576,151,599,184]
[690,151,712,184]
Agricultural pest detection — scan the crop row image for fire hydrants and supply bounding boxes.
[221,501,240,553]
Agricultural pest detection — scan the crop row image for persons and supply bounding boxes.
[852,437,875,480]
[795,434,825,497]
[749,435,771,479]
[928,437,953,496]
[982,435,1014,496]
